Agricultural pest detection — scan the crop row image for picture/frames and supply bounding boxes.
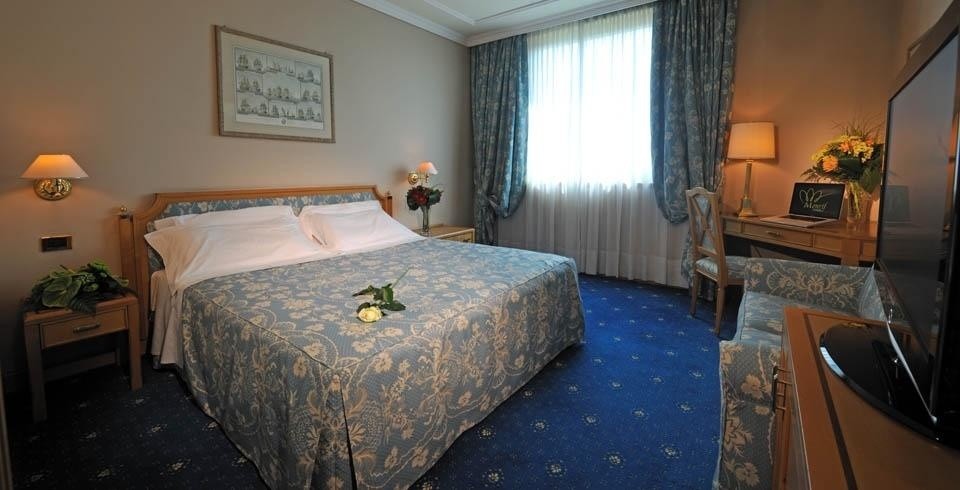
[214,24,335,144]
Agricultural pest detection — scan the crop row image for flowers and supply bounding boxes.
[799,113,884,211]
[352,267,410,323]
[405,185,444,211]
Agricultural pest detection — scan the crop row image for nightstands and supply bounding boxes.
[413,226,476,243]
[22,291,142,420]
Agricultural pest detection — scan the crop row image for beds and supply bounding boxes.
[119,185,585,490]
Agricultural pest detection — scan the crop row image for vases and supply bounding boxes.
[847,182,867,223]
[421,206,431,235]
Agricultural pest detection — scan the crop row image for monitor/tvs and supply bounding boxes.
[817,2,959,454]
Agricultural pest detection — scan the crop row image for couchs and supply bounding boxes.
[712,257,886,488]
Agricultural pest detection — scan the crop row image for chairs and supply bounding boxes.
[685,187,748,334]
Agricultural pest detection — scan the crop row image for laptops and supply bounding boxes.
[760,182,846,228]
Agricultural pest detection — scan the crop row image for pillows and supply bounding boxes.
[153,214,199,230]
[144,205,325,296]
[299,200,425,256]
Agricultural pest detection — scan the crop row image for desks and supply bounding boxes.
[721,216,877,267]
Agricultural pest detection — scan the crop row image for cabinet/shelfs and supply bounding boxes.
[770,307,959,489]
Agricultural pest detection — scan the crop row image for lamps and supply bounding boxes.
[408,162,438,185]
[20,154,89,201]
[726,122,776,217]
